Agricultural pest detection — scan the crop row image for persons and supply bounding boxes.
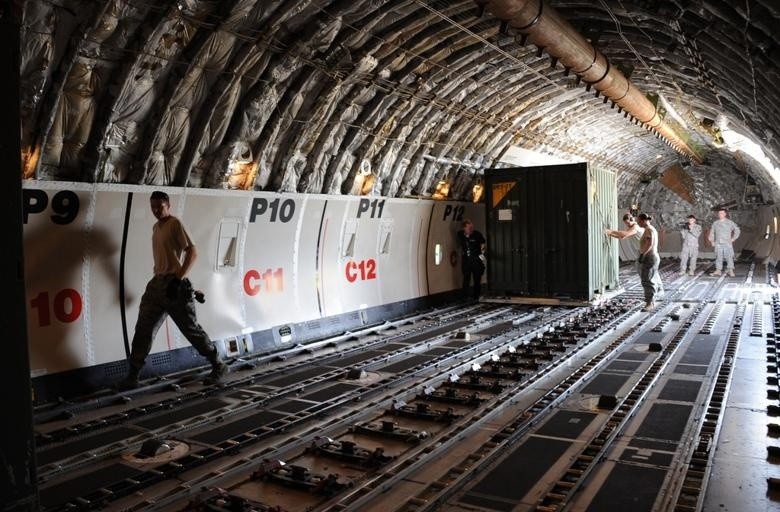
[708,209,741,277]
[678,215,702,276]
[455,219,486,304]
[606,213,664,297]
[112,190,228,394]
[636,213,660,311]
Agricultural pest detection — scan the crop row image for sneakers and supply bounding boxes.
[676,270,695,277]
[727,269,736,278]
[641,301,656,313]
[708,268,722,277]
[202,361,231,385]
[109,377,140,392]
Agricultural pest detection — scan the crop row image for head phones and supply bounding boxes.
[630,217,634,221]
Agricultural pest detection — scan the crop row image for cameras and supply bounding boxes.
[683,222,690,230]
